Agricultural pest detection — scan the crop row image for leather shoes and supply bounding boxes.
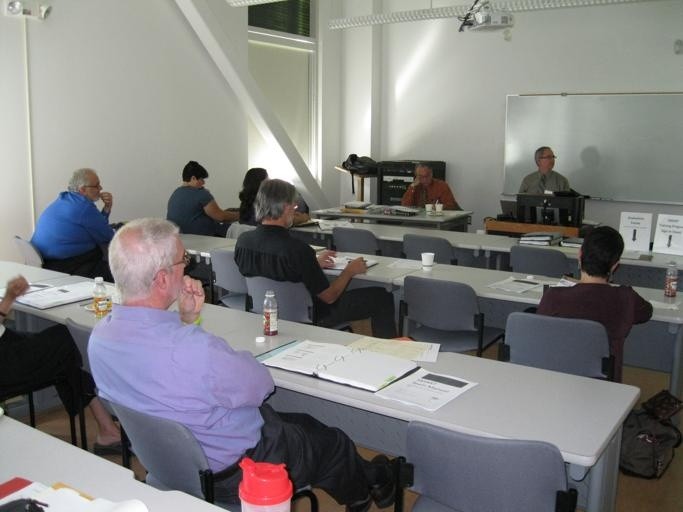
[374,455,407,506]
[343,455,386,512]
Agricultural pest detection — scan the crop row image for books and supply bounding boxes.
[520,232,586,248]
[315,253,380,272]
[258,338,422,391]
[375,366,480,412]
[1,282,94,311]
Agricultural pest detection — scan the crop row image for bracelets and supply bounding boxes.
[0,311,8,318]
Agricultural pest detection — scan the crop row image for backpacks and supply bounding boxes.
[341,153,377,194]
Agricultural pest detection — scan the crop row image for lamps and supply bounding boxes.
[0,0,54,22]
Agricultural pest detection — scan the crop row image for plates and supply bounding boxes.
[86,301,112,311]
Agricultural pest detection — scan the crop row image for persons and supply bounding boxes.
[518,147,571,194]
[166,161,242,237]
[401,164,460,211]
[535,226,652,382]
[29,168,114,283]
[238,168,310,226]
[0,275,134,456]
[233,179,397,339]
[86,217,398,512]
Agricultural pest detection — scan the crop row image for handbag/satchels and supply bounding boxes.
[619,406,680,479]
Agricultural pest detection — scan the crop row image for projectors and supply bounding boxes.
[467,12,515,31]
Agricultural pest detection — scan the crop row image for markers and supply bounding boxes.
[590,197,601,201]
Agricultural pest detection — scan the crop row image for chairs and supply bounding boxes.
[1,194,681,511]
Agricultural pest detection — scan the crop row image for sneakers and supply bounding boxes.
[93,440,133,457]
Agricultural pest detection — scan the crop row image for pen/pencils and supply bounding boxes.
[348,260,368,263]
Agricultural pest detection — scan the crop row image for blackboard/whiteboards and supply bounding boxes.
[500,92,683,206]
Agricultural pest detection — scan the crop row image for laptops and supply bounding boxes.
[500,200,517,218]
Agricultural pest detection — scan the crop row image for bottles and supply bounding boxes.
[262,291,277,336]
[664,261,678,297]
[92,277,107,319]
[236,454,293,511]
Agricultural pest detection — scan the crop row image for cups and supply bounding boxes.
[435,204,442,216]
[424,204,433,215]
[421,253,434,270]
